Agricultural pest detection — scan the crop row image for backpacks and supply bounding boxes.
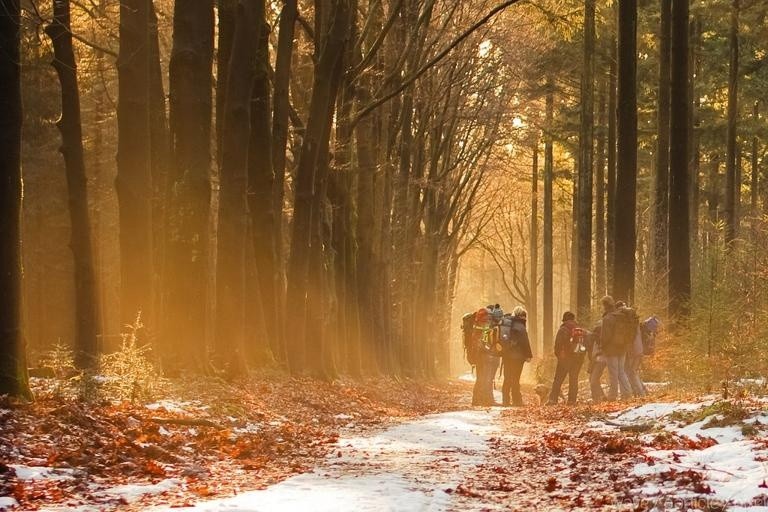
[614,306,639,349]
[491,316,514,355]
[639,315,660,355]
[466,327,484,364]
[567,327,587,356]
[460,311,477,349]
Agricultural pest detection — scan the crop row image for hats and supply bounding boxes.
[560,311,575,322]
[491,304,504,318]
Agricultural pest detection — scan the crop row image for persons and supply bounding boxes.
[473,295,646,407]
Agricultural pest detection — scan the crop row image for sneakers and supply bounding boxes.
[544,400,578,407]
